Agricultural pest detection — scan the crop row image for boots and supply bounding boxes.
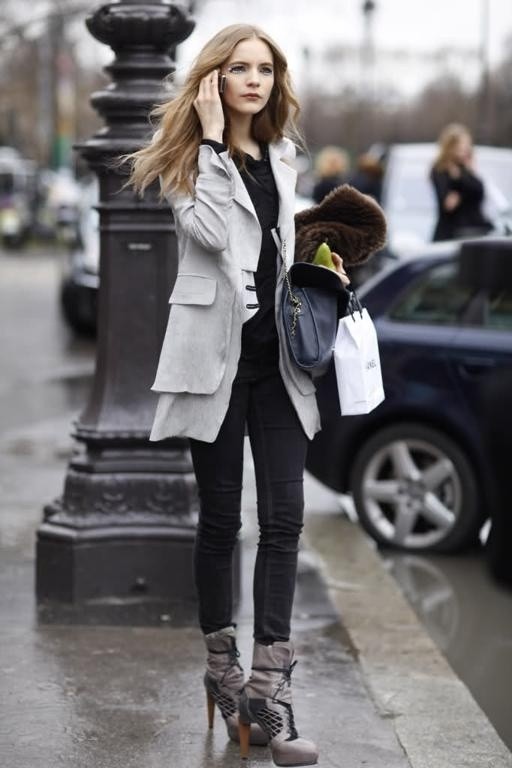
[238,640,320,766]
[203,622,271,747]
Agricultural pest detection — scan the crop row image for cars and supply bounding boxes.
[303,233,512,555]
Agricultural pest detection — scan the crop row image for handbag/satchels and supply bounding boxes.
[279,261,351,382]
[333,289,387,418]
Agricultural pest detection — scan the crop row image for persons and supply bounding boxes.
[313,144,350,205]
[353,142,385,206]
[116,23,358,767]
[430,124,493,240]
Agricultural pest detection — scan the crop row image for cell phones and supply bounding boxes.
[211,73,226,93]
